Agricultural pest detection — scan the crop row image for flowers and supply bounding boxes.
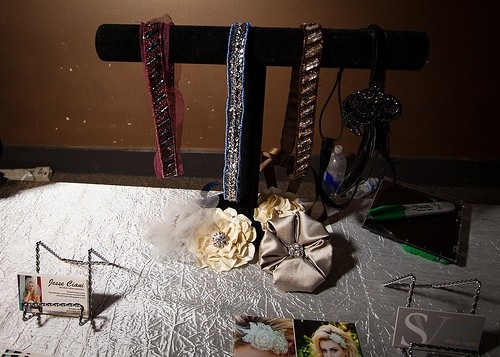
[330,332,347,349]
[242,322,289,354]
[148,187,332,291]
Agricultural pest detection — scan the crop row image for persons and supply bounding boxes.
[23,278,41,303]
[233,315,295,357]
[312,325,358,357]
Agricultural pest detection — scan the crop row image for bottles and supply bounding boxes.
[322,145,347,192]
[346,178,379,200]
